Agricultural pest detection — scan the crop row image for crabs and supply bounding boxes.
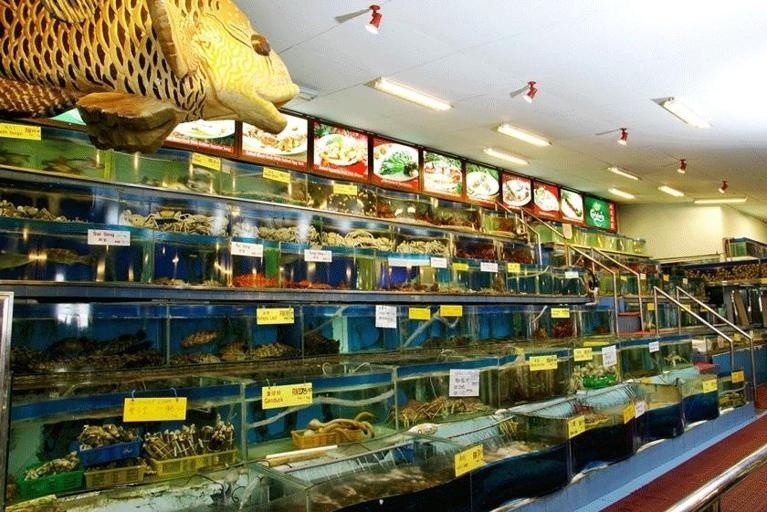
[123,211,227,238]
[386,405,425,427]
[322,229,393,253]
[416,396,467,419]
[397,240,448,257]
[232,221,322,246]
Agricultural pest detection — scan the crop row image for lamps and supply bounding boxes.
[694,198,746,205]
[373,77,454,113]
[482,147,530,167]
[678,159,687,174]
[366,4,383,35]
[657,185,684,197]
[609,165,642,182]
[606,188,634,200]
[618,128,629,146]
[718,179,729,193]
[523,80,538,102]
[498,124,551,149]
[661,96,711,130]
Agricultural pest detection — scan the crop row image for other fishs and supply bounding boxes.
[0,1,301,154]
